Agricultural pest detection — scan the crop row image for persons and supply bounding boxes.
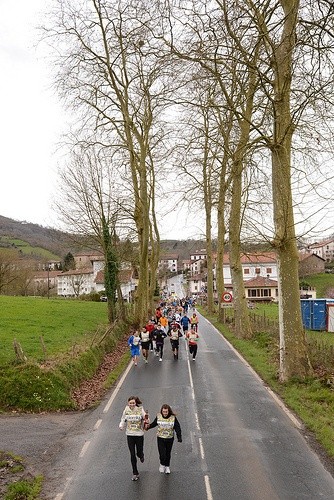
[146,404,182,474]
[128,283,199,366]
[118,395,151,481]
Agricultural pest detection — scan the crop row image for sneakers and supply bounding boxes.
[140,457,144,462]
[164,466,170,473]
[159,463,165,473]
[131,472,140,481]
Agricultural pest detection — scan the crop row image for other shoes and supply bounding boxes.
[143,357,146,360]
[134,362,137,366]
[156,353,159,357]
[158,358,162,361]
[132,358,135,361]
[177,353,178,359]
[174,354,175,360]
[193,358,195,360]
[145,359,148,363]
[150,349,153,353]
